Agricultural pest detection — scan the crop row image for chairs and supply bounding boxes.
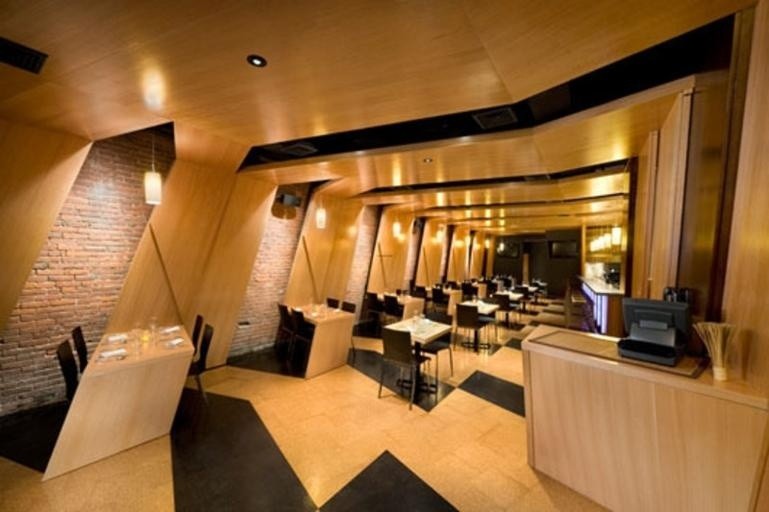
[268,274,549,411]
[55,315,215,409]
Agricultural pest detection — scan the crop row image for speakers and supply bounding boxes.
[0,36,50,75]
[280,193,296,205]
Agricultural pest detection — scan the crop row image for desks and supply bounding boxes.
[39,323,197,484]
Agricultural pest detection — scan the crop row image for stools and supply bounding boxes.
[564,278,594,332]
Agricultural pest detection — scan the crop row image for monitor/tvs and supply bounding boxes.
[495,238,522,259]
[616,298,689,367]
[550,239,578,258]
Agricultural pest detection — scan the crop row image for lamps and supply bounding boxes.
[143,128,163,206]
